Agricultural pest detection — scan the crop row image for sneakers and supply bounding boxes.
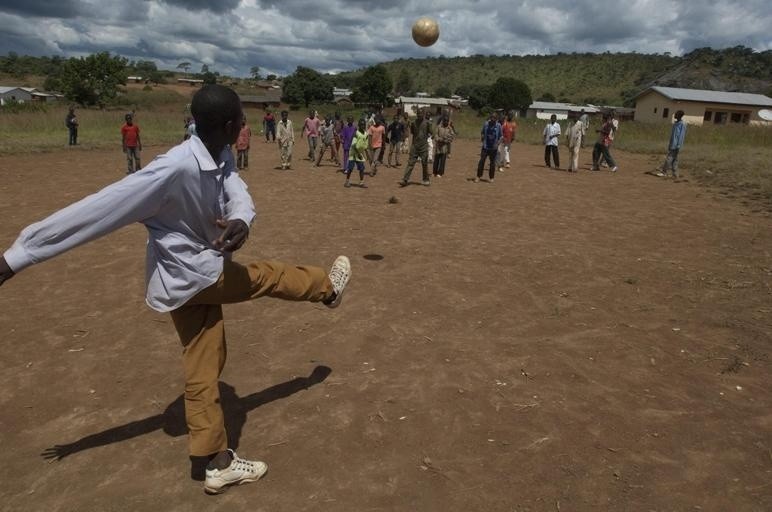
[589,163,617,172]
[655,172,678,179]
[544,163,578,173]
[323,254,353,308]
[421,181,430,186]
[432,173,440,178]
[309,154,348,173]
[359,181,369,189]
[368,161,402,176]
[273,163,294,170]
[343,180,350,188]
[473,177,494,184]
[395,180,408,186]
[498,163,511,173]
[203,447,269,496]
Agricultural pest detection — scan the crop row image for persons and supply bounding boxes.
[65,107,79,145]
[261,109,295,169]
[543,108,618,171]
[301,106,455,185]
[184,118,195,139]
[474,110,518,182]
[656,110,686,178]
[121,114,142,173]
[0,85,351,495]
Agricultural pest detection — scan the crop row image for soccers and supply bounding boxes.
[412,19,440,46]
